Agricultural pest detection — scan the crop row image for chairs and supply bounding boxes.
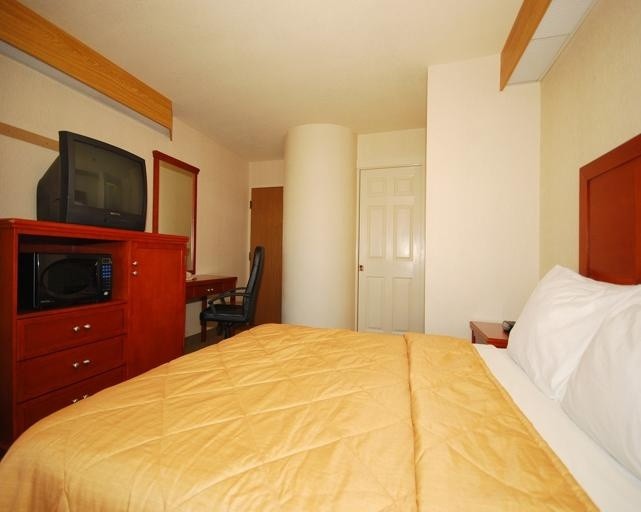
[198,245,264,343]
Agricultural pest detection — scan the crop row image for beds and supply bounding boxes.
[1,132,640,511]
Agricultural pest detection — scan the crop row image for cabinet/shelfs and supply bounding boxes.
[1,216,190,456]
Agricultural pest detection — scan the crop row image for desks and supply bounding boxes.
[186,273,239,344]
[467,319,517,350]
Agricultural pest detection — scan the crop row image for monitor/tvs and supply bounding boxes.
[36,131,147,231]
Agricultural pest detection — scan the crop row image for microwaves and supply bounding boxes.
[17,250,113,313]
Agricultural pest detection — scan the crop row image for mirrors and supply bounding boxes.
[150,149,200,276]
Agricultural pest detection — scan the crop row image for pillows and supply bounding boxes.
[506,263,641,404]
[561,291,640,483]
